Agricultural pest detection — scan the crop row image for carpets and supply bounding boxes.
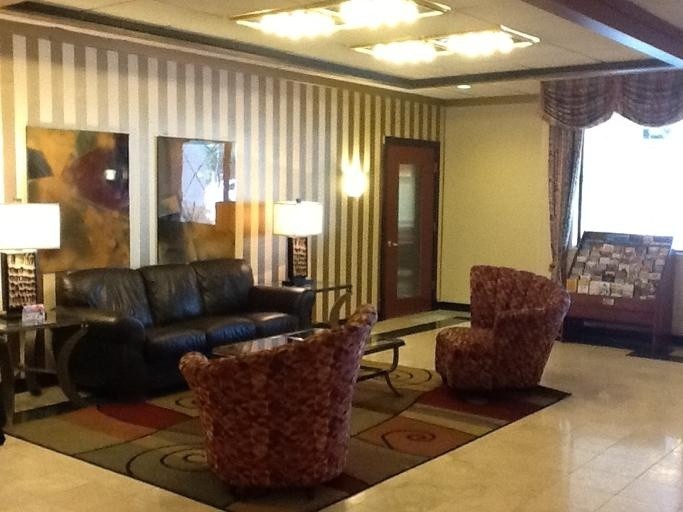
[0,358,572,511]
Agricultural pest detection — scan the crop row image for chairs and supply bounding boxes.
[434,261,575,401]
[175,302,384,491]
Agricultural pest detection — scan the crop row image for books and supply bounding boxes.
[563,232,672,300]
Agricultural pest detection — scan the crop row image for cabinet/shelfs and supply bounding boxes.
[564,231,677,355]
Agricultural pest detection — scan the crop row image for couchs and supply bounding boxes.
[54,257,317,405]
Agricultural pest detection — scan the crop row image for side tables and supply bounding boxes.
[0,307,95,426]
[280,282,354,328]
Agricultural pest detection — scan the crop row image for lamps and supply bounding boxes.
[0,200,63,317]
[271,198,324,285]
